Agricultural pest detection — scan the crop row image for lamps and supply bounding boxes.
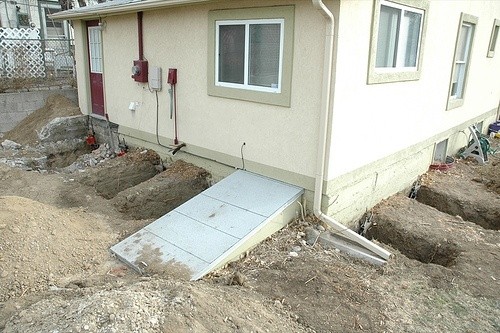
[98,23,104,31]
